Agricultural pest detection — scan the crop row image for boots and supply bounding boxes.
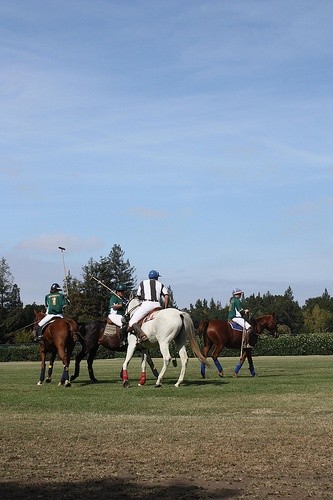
[120,328,128,347]
[33,324,43,343]
[131,323,148,343]
[242,328,254,349]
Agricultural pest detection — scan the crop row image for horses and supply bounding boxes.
[198,314,279,379]
[33,307,78,388]
[69,291,215,388]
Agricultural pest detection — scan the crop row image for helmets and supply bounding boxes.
[148,270,162,278]
[115,285,127,290]
[232,289,242,295]
[51,283,62,291]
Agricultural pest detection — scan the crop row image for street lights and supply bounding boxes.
[57,246,70,297]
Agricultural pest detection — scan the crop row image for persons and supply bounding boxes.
[129,269,168,343]
[33,283,71,342]
[108,285,128,348]
[228,288,254,348]
